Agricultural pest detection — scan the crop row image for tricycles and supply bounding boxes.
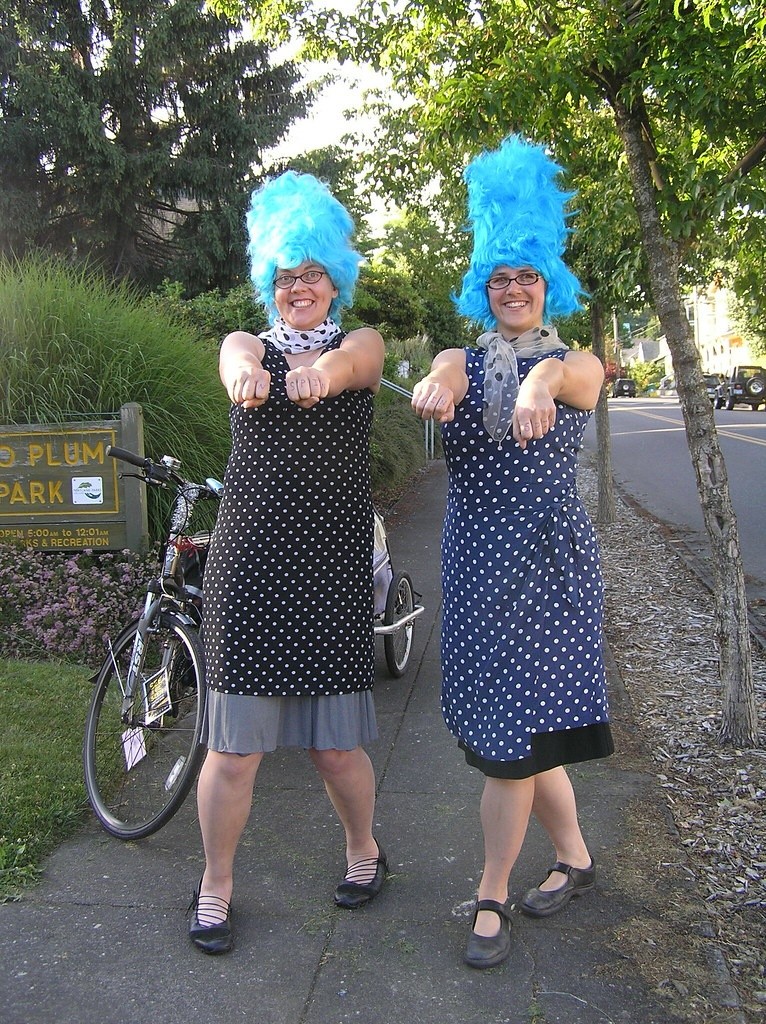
[83,445,426,843]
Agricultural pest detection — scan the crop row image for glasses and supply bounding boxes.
[485,273,544,290]
[273,271,327,289]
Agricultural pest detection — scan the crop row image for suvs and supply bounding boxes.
[713,365,766,411]
[613,378,636,398]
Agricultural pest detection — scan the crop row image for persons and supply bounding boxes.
[184,172,390,955]
[412,133,616,968]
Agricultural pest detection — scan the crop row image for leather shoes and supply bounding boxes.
[519,855,596,918]
[464,895,514,969]
[186,876,234,954]
[334,836,389,909]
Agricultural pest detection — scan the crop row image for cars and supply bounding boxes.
[702,374,728,407]
